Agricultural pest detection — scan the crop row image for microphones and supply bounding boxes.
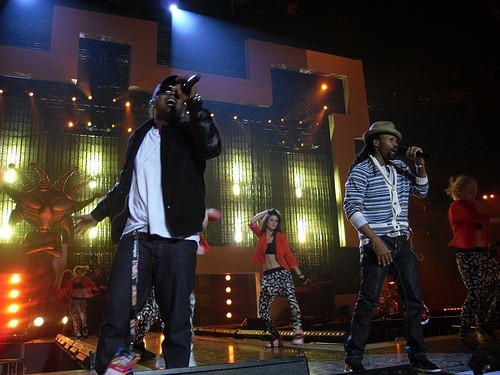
[399,145,426,158]
[174,74,200,100]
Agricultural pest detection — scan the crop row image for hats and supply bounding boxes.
[362,121,403,144]
[151,74,187,99]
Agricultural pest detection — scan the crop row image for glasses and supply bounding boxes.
[160,85,172,93]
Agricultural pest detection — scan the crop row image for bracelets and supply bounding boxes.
[298,272,303,277]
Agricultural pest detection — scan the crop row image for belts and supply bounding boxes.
[386,230,406,238]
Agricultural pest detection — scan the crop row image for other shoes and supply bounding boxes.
[462,341,483,351]
[479,324,494,341]
[265,338,283,347]
[133,342,157,360]
[410,355,441,373]
[293,331,303,344]
[344,363,368,375]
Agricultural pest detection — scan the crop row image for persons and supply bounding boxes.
[342,119,441,375]
[73,72,222,375]
[445,177,500,350]
[56,265,107,340]
[248,208,310,348]
[132,208,209,369]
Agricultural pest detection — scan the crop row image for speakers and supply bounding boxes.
[133,354,310,375]
[241,318,263,330]
